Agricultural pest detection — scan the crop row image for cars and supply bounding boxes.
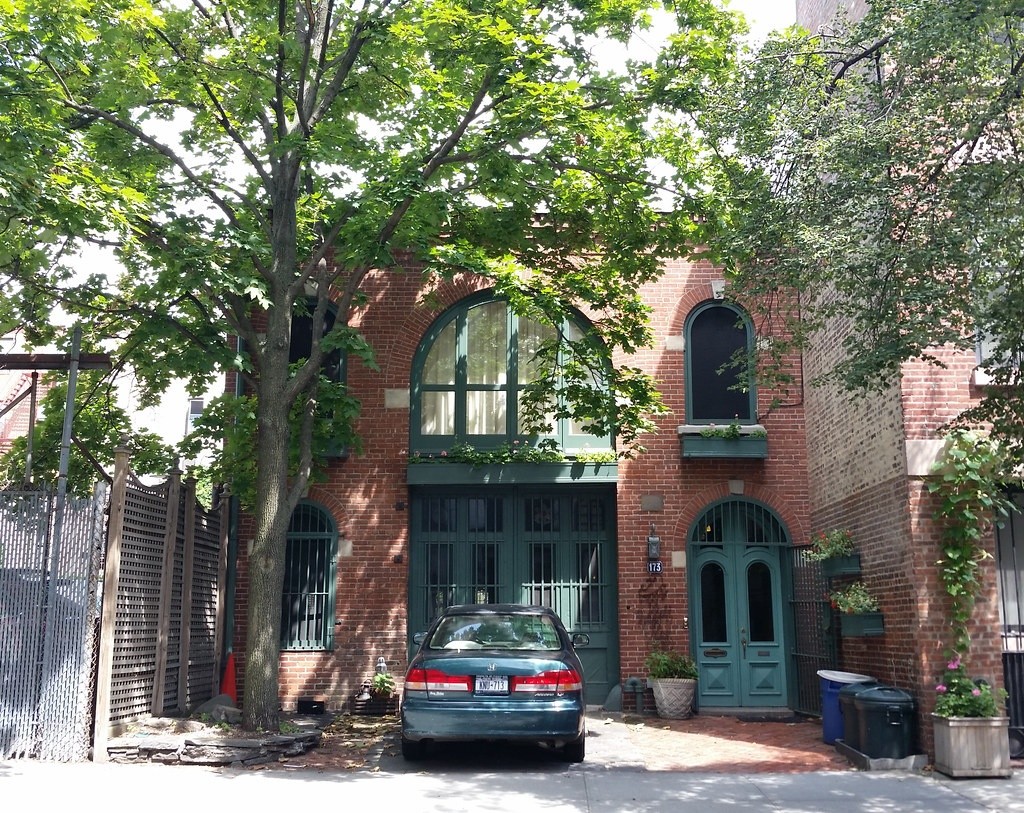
[401,604,590,763]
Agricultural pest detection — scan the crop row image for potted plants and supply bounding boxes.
[642,645,698,719]
[347,672,400,717]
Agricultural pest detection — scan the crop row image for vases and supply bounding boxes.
[822,554,860,577]
[930,713,1015,779]
[841,613,884,637]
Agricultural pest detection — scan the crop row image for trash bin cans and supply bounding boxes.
[838,684,885,749]
[816,669,879,745]
[854,684,916,759]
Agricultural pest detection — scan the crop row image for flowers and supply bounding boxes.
[830,582,878,613]
[709,414,740,426]
[801,528,853,562]
[399,440,528,462]
[936,649,981,696]
